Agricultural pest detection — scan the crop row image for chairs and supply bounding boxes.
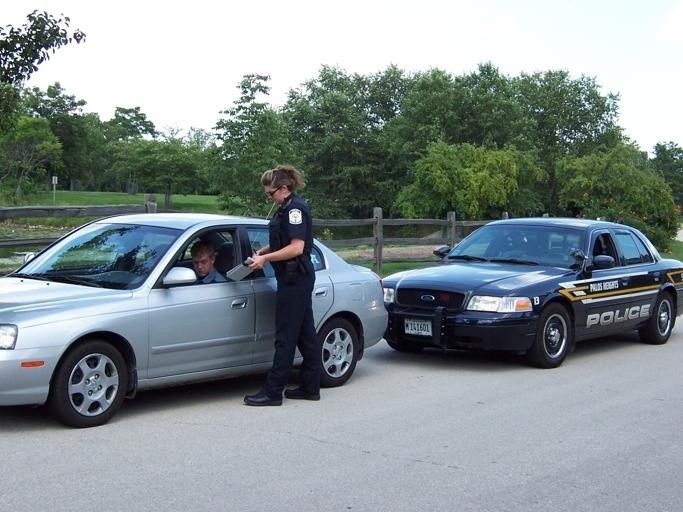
[215,241,235,281]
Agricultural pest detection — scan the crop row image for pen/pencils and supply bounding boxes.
[253,249,257,254]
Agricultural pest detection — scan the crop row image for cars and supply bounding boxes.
[0,212,389,428]
[382,216,683,368]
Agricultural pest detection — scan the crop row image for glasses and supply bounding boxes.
[264,183,285,199]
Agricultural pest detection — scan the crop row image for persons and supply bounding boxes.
[243,164,321,406]
[189,240,227,283]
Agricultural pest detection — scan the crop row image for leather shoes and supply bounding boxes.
[244,387,321,406]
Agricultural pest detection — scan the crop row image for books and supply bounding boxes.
[226,256,253,281]
